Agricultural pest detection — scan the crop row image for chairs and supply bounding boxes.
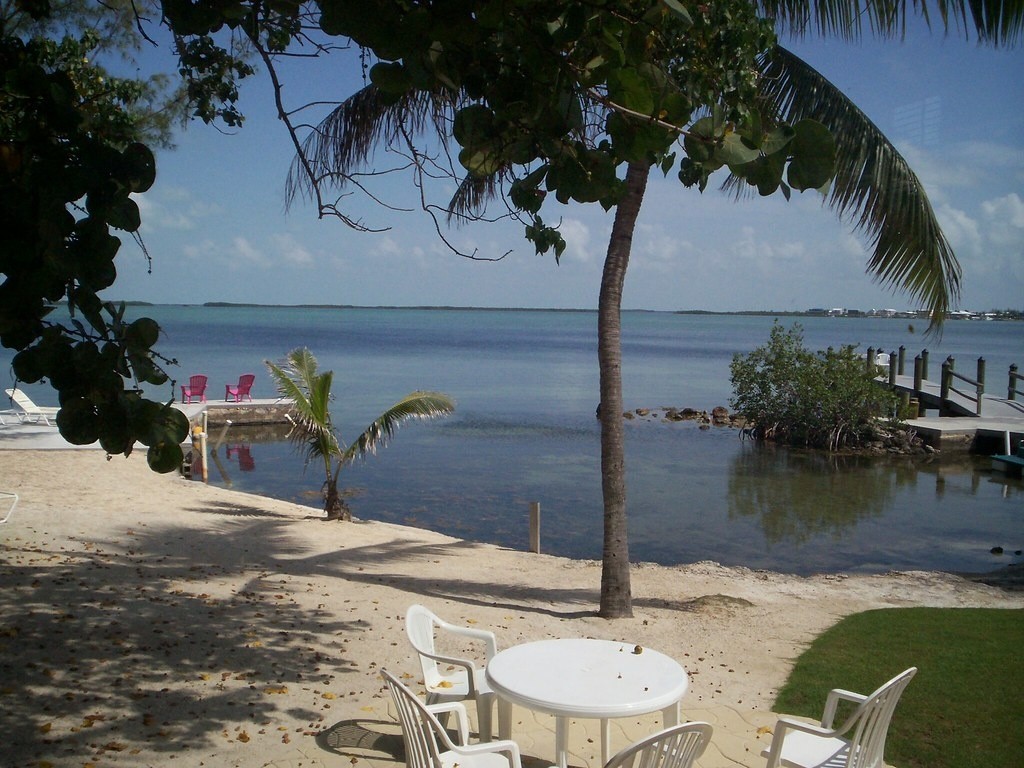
[226,442,254,471]
[181,375,208,404]
[404,604,497,744]
[379,669,522,768]
[760,667,918,768]
[548,721,713,768]
[0,389,58,426]
[225,374,255,403]
[875,353,890,373]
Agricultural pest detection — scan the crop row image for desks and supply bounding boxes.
[484,638,689,768]
